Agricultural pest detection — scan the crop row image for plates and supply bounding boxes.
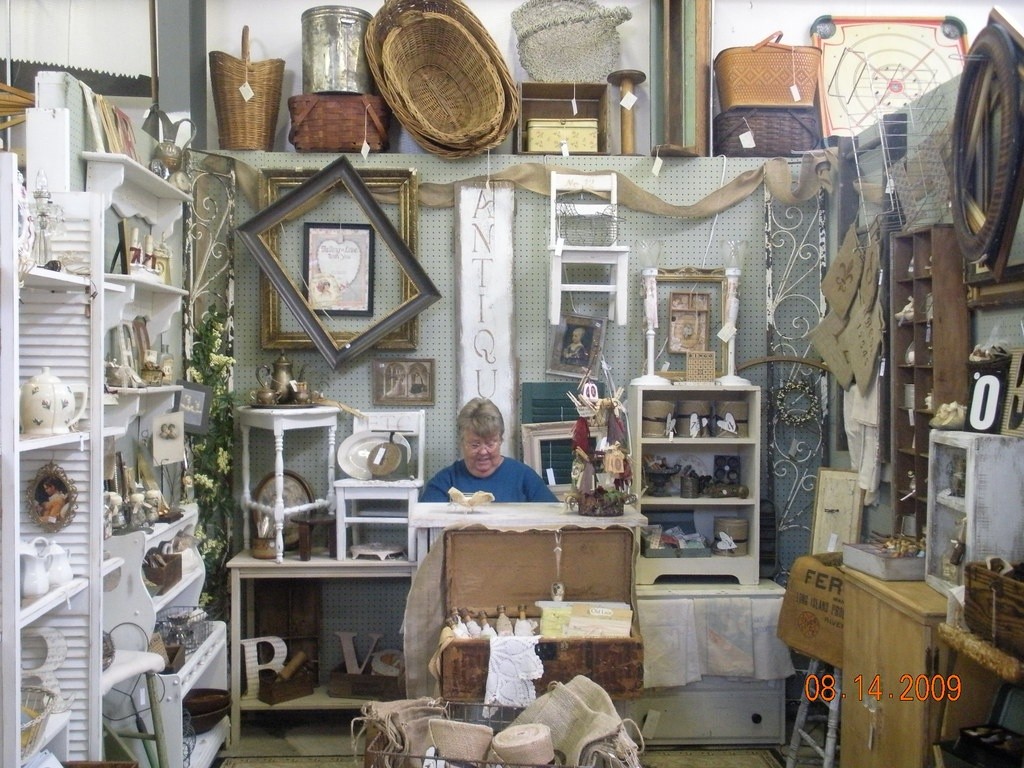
[337,430,411,480]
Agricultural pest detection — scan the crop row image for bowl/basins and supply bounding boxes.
[182,689,233,737]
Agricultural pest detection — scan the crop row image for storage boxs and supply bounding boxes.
[257,669,314,706]
[842,543,924,580]
[526,118,598,152]
[328,672,406,702]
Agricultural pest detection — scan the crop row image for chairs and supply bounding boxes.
[333,409,424,562]
[547,170,630,326]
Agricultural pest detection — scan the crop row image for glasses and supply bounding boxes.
[463,438,500,448]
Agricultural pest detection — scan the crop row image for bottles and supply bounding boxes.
[444,604,533,639]
[158,344,174,385]
[160,612,206,650]
[141,362,163,387]
[104,489,159,539]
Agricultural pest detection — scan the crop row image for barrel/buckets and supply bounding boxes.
[301,5,374,96]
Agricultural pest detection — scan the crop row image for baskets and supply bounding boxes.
[156,605,216,657]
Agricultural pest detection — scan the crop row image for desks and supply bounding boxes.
[225,551,417,746]
[236,406,336,563]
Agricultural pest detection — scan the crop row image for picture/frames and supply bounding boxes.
[233,155,443,374]
[523,420,597,480]
[809,466,866,556]
[545,313,608,380]
[641,265,728,382]
[810,14,971,147]
[80,81,141,164]
[371,357,436,408]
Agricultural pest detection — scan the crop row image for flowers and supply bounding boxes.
[187,304,234,619]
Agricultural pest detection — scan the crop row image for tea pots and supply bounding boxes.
[19,367,88,434]
[19,537,73,598]
[162,531,198,574]
[256,348,310,403]
[154,109,197,182]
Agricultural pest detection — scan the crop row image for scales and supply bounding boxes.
[367,430,414,481]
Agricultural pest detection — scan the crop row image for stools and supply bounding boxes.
[291,514,337,561]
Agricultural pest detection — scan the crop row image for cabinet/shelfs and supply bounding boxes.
[441,528,643,702]
[633,579,788,746]
[0,143,91,768]
[513,79,612,156]
[838,565,1000,768]
[889,224,968,543]
[80,151,228,768]
[628,384,761,585]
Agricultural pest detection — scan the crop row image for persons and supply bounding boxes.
[159,424,177,439]
[561,327,589,367]
[35,477,66,524]
[418,398,561,502]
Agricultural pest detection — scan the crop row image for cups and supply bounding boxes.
[681,476,699,498]
[289,391,312,403]
[251,537,287,559]
[250,381,283,404]
[905,383,914,408]
